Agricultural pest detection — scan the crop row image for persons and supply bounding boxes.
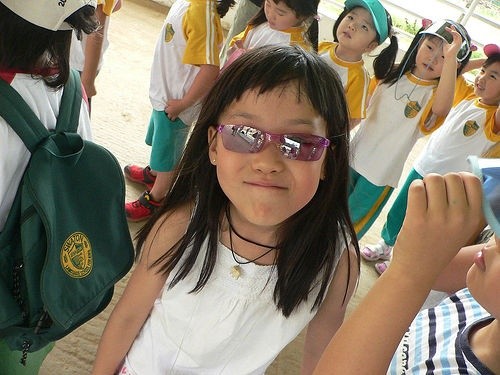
[313,155,500,375]
[0,0,91,375]
[337,18,478,241]
[229,0,321,54]
[124,0,237,221]
[69,0,122,119]
[318,0,398,131]
[361,44,500,275]
[92,44,361,375]
[219,0,264,70]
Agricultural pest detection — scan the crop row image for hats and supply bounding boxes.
[466,154,499,241]
[344,0,388,45]
[483,42,500,56]
[0,0,97,32]
[420,19,470,60]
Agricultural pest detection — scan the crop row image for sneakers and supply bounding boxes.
[124,165,173,222]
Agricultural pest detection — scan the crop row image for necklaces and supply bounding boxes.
[226,201,283,280]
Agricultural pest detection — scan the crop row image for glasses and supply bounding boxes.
[215,121,330,162]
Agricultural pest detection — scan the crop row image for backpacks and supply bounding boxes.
[0,67,135,367]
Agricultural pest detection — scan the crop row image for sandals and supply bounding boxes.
[362,239,391,275]
